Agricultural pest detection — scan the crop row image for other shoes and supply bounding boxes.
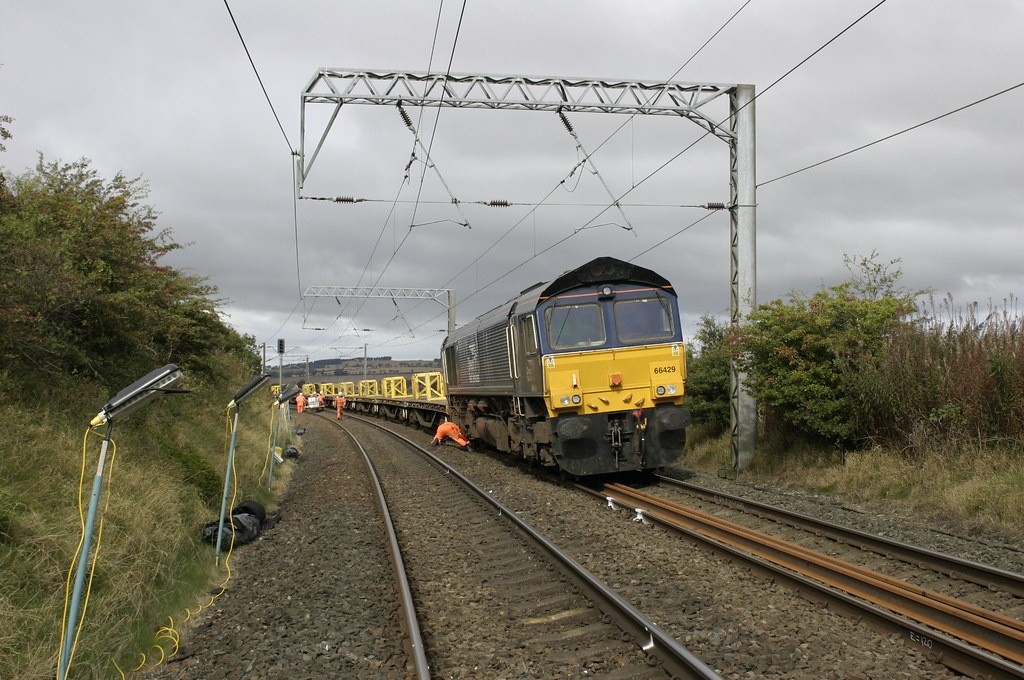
[338,418,341,420]
[466,446,475,452]
[432,438,438,446]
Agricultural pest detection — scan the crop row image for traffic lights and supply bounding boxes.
[277,339,285,353]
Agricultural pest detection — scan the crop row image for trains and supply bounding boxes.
[270,255,691,483]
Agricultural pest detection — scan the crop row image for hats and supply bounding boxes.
[313,391,316,394]
[300,393,303,396]
[338,392,343,396]
[320,391,323,394]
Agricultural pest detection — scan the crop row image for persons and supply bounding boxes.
[334,392,346,420]
[311,391,325,412]
[431,422,474,452]
[295,393,306,413]
